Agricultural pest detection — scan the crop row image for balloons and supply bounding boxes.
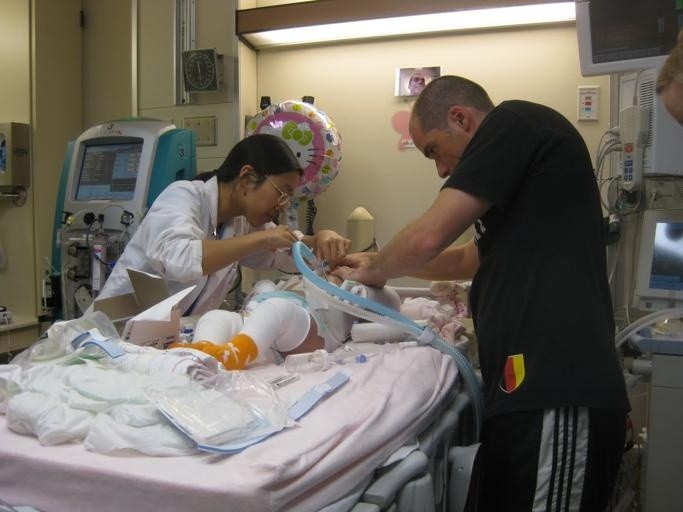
[244,100,343,202]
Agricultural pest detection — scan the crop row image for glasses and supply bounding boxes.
[264,173,291,211]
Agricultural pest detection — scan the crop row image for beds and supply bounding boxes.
[0,285,482,512]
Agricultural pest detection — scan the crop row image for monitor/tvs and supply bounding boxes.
[633,207,683,311]
[576,1,683,77]
[62,118,176,225]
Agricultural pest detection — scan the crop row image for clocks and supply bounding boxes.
[181,47,220,94]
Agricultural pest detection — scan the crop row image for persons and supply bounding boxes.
[408,73,426,95]
[329,74,634,512]
[82,133,352,318]
[168,265,402,370]
[655,30,683,128]
